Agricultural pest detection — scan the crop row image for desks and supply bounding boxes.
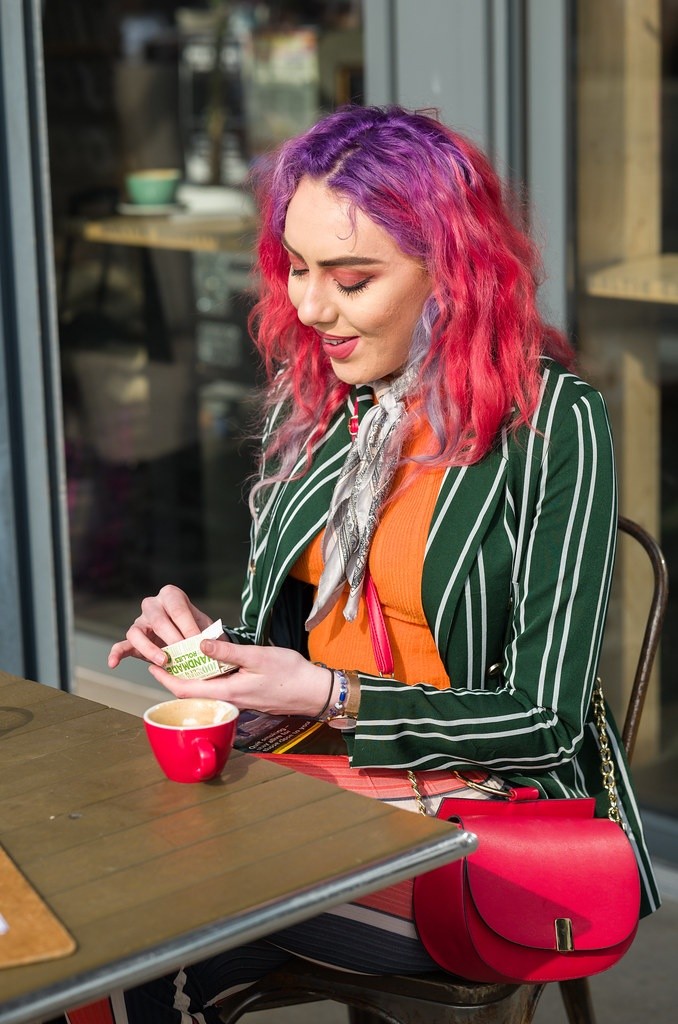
[0,670,480,1024]
[84,217,255,578]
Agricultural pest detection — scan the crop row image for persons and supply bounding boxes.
[179,0,264,188]
[106,98,660,1024]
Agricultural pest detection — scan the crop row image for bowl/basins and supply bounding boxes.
[125,168,184,206]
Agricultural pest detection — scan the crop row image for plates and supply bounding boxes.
[112,202,190,215]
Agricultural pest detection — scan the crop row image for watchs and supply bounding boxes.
[328,669,361,730]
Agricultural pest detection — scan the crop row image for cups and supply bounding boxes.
[142,698,239,784]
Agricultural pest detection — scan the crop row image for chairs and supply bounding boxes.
[202,513,668,1024]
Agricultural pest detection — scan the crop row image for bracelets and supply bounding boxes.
[307,660,350,724]
